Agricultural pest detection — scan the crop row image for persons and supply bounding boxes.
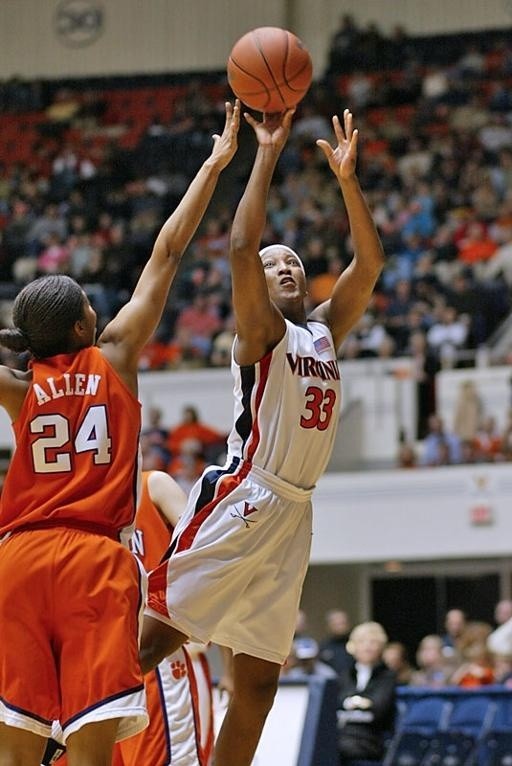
[54,470,216,766]
[280,597,512,687]
[43,106,386,766]
[0,13,512,463]
[0,98,240,766]
[331,620,397,766]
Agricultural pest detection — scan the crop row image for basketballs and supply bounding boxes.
[227,26,312,113]
